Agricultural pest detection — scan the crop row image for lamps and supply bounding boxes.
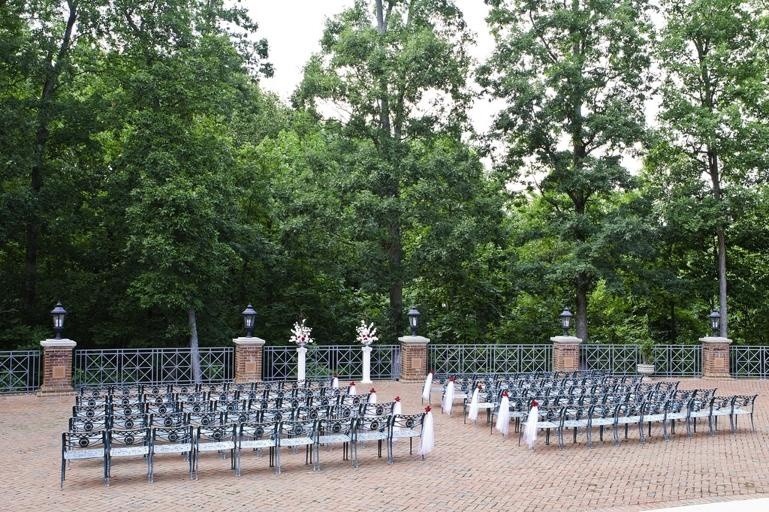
[49,296,67,340]
[558,304,574,336]
[239,299,257,338]
[405,303,420,335]
[707,304,722,333]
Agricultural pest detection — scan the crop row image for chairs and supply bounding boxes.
[346,414,392,468]
[731,393,757,432]
[109,427,150,485]
[379,412,426,464]
[310,417,354,471]
[66,376,400,470]
[518,405,560,449]
[712,396,735,436]
[561,405,589,446]
[643,400,667,442]
[422,369,718,436]
[233,420,279,477]
[589,404,617,444]
[150,425,194,485]
[615,402,643,444]
[61,431,108,484]
[192,423,237,480]
[689,397,714,438]
[272,419,316,473]
[666,399,690,441]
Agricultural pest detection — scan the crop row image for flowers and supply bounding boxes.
[287,317,381,346]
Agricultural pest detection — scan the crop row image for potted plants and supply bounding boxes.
[635,337,659,378]
